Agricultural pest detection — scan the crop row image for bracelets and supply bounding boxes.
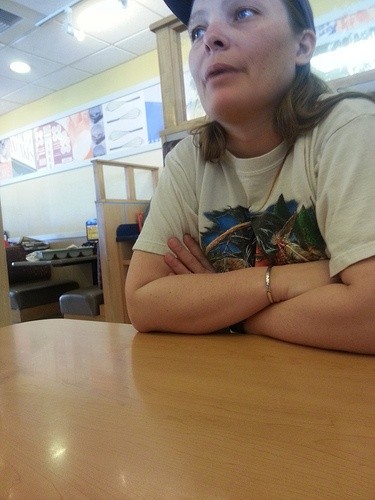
[229,320,247,334]
[265,265,274,304]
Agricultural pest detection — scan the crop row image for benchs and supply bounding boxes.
[59,286,103,321]
[6,245,81,322]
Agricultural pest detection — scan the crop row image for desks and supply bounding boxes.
[1,318,374,498]
[12,254,99,286]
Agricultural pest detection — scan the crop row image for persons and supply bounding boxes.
[124,0,375,356]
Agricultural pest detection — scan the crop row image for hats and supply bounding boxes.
[163,0,315,31]
